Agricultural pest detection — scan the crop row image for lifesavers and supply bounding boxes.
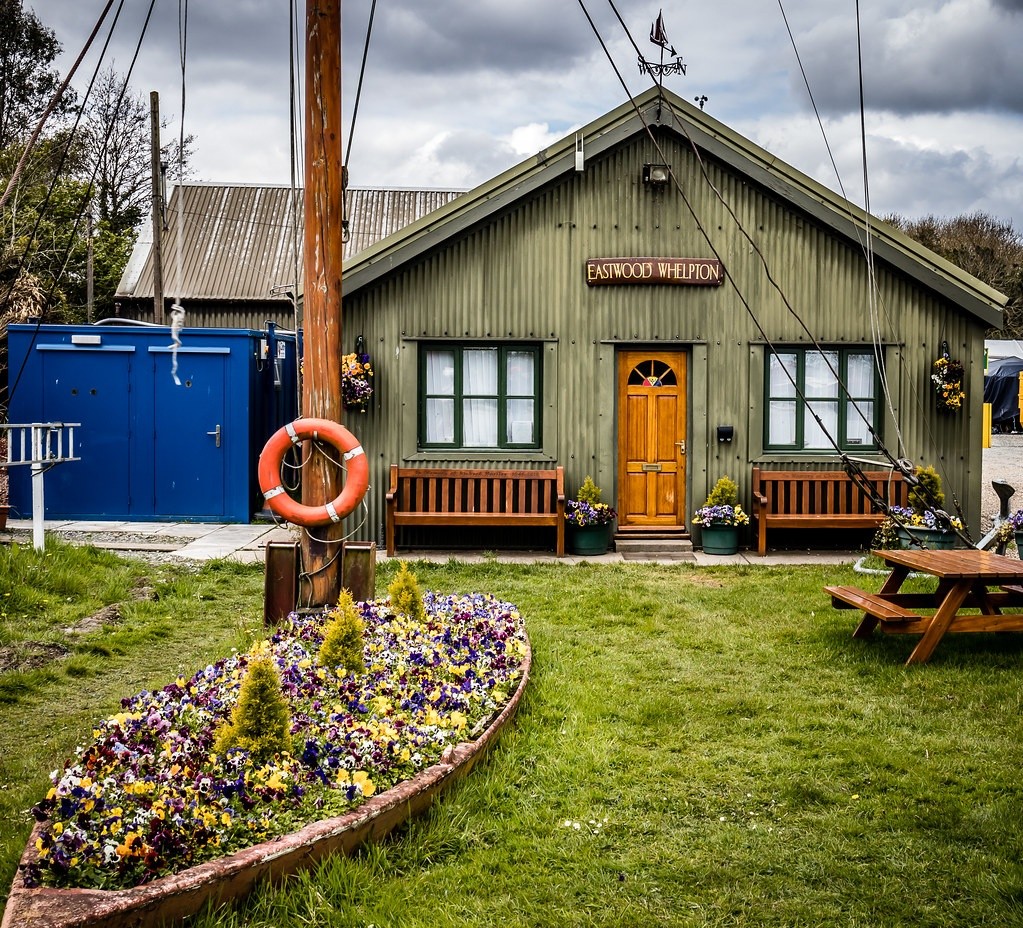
[256,416,370,529]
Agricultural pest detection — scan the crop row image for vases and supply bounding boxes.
[703,526,739,555]
[1014,534,1023,560]
[897,528,960,550]
[574,525,609,555]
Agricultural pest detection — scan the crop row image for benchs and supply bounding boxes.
[386,465,564,556]
[753,468,919,557]
[822,586,925,665]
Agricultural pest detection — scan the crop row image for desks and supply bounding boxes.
[871,549,1023,575]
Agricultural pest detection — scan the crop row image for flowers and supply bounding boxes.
[693,477,750,526]
[565,477,613,526]
[932,354,965,411]
[871,505,964,548]
[299,353,373,416]
[999,511,1023,543]
